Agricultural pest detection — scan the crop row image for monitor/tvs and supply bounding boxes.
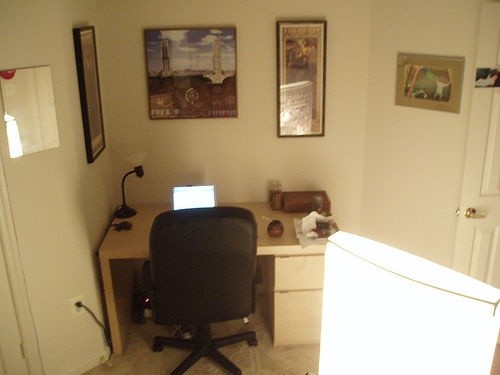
[171,184,217,210]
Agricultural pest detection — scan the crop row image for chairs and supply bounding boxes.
[151,206,264,375]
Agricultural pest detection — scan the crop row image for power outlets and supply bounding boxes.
[71,295,86,316]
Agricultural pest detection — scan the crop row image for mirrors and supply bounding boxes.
[0,64,60,158]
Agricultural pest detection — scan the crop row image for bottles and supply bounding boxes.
[267,221,283,237]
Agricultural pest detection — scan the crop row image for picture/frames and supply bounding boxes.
[143,27,239,120]
[395,52,465,114]
[73,25,106,164]
[276,20,328,137]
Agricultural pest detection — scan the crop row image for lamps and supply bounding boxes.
[113,165,144,218]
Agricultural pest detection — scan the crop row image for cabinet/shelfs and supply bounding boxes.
[98,206,336,355]
